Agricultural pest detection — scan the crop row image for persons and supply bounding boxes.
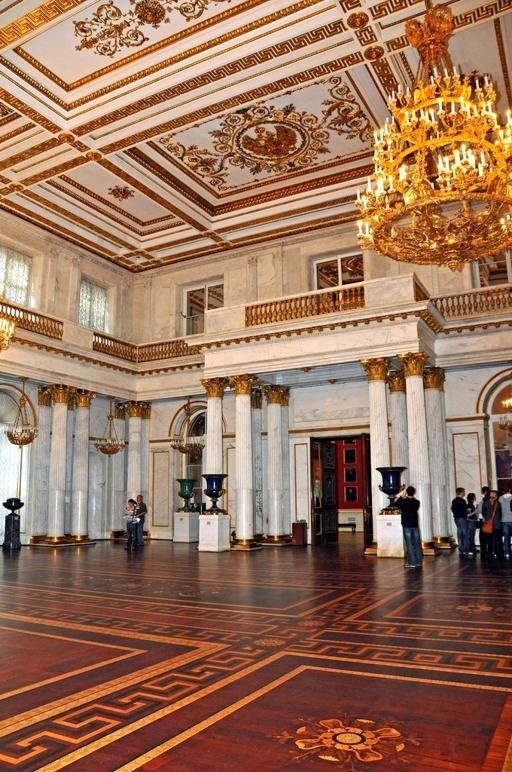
[124,495,148,551]
[392,486,424,568]
[451,486,512,568]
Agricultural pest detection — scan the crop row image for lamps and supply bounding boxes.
[498,397,512,433]
[356,4,512,272]
[4,377,37,449]
[94,395,125,458]
[170,396,204,453]
[0,319,15,350]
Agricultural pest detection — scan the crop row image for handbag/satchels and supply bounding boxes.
[122,515,140,524]
[482,518,493,533]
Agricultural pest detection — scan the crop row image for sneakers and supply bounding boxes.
[404,562,422,567]
[458,548,479,556]
[124,543,144,551]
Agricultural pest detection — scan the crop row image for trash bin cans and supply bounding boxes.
[292,523,307,545]
